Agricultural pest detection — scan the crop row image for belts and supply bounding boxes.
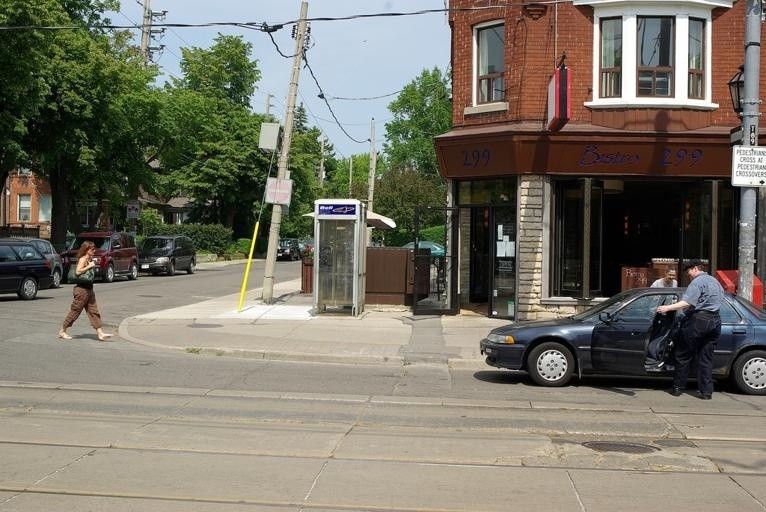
[694,310,719,316]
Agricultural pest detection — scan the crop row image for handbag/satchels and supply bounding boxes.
[72,278,93,289]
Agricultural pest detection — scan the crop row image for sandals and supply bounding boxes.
[58,330,73,340]
[98,334,114,341]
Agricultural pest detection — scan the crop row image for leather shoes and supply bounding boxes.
[696,391,712,399]
[671,388,681,396]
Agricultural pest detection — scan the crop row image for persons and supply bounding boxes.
[650,265,678,288]
[656,260,725,400]
[58,241,114,341]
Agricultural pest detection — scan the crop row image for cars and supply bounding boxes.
[481,288,766,391]
[401,241,445,268]
[1,231,197,300]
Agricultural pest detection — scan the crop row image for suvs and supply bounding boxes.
[277,238,300,260]
